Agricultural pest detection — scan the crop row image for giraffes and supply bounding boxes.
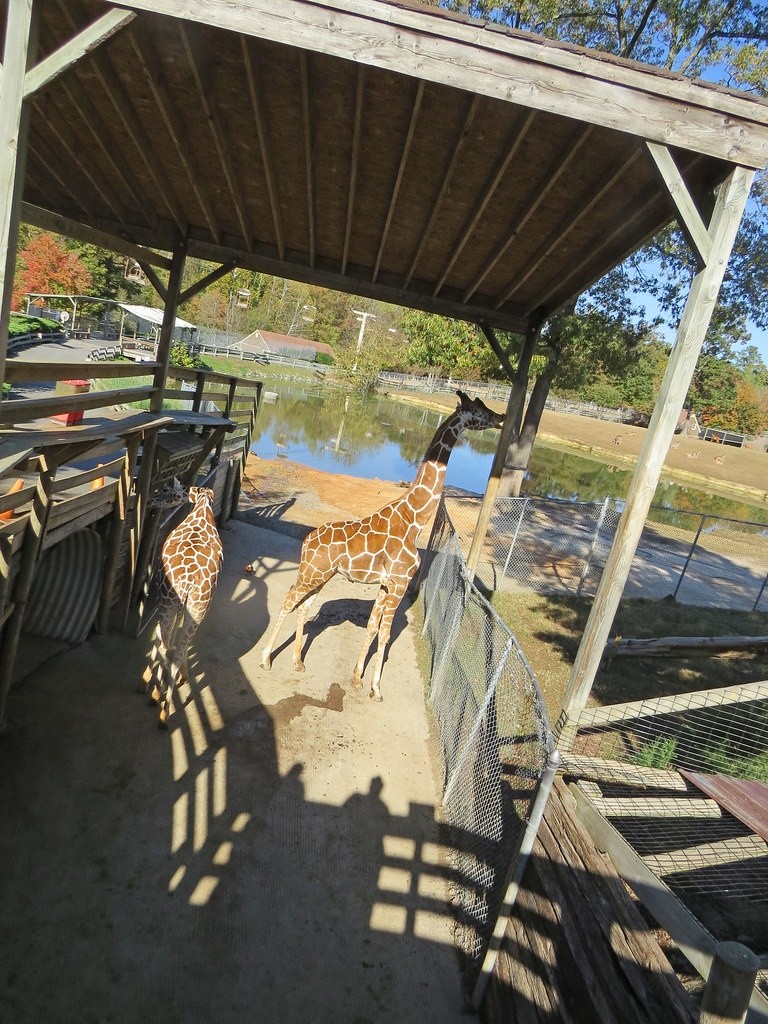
[261,389,507,702]
[136,487,224,729]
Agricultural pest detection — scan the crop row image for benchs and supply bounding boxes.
[88,346,123,361]
